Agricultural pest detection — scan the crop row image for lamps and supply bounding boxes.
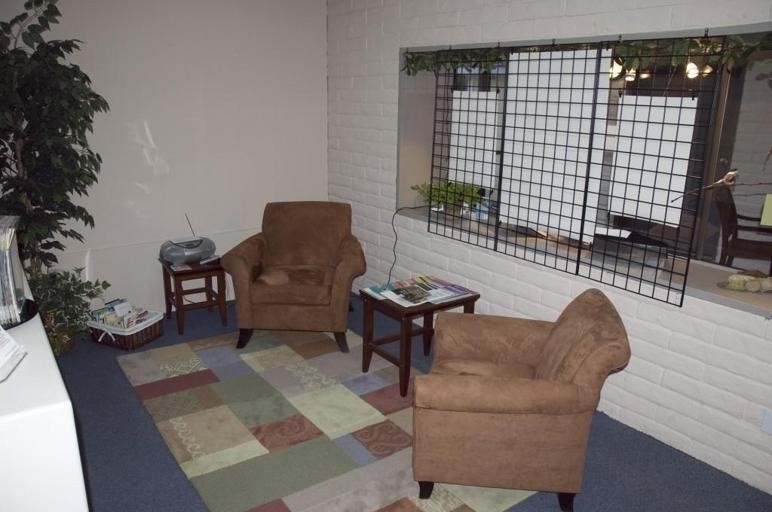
[609,56,715,83]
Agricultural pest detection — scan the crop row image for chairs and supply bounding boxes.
[412,287,630,512]
[714,186,772,275]
[220,200,367,353]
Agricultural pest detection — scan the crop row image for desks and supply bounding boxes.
[760,191,772,227]
[0,263,92,511]
[359,277,480,396]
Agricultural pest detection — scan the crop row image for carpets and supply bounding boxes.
[116,327,542,512]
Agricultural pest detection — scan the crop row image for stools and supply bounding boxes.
[159,258,228,334]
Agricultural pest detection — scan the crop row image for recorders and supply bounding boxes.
[160,236,217,267]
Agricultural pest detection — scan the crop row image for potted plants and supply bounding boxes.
[0,1,110,356]
[410,178,492,220]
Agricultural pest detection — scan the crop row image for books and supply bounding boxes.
[91,297,150,328]
[362,274,475,308]
